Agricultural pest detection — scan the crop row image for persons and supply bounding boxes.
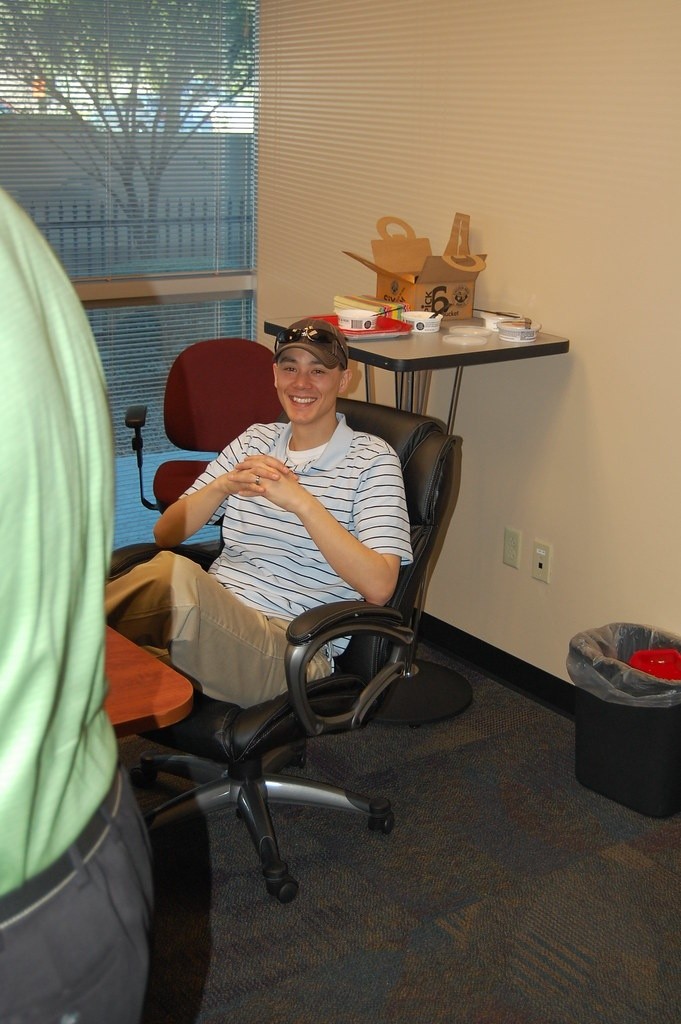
[0,190,418,1024]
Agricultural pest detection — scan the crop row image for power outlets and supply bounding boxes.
[502,524,521,567]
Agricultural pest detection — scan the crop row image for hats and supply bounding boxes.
[272,318,348,371]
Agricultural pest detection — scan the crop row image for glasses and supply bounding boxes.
[274,328,348,370]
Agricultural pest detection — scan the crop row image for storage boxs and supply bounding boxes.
[341,210,488,321]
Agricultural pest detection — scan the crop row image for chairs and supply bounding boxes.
[109,399,458,898]
[126,338,274,527]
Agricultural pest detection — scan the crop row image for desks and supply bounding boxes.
[263,316,570,727]
[103,624,193,741]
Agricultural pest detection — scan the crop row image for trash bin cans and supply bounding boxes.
[569,622,681,818]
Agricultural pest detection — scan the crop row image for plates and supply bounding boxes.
[308,315,414,340]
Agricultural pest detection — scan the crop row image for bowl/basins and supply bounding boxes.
[338,309,379,330]
[497,319,542,342]
[401,311,443,333]
[481,312,522,332]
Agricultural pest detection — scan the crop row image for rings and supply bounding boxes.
[256,475,260,485]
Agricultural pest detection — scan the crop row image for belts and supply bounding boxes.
[0,770,123,924]
